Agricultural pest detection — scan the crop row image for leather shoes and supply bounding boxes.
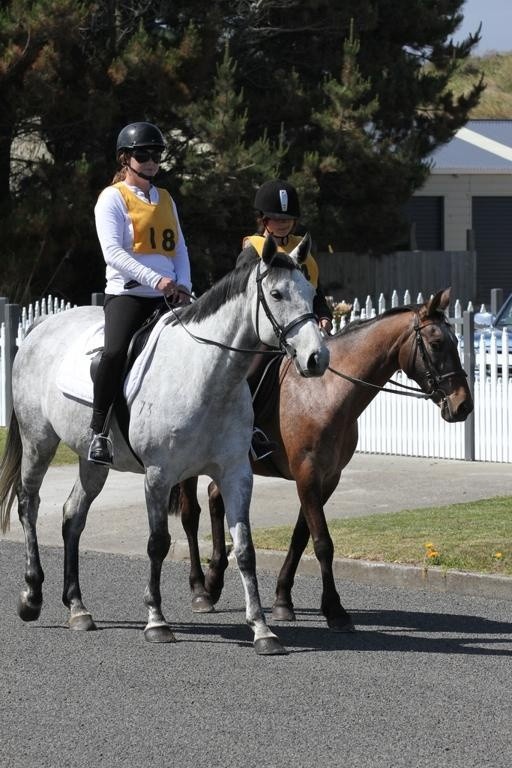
[89,431,111,461]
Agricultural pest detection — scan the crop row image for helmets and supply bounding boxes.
[116,122,168,150]
[253,179,299,220]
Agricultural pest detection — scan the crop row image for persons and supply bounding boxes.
[86,120,194,464]
[233,177,334,464]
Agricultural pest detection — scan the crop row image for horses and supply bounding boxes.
[0,230,331,656]
[168,286,475,633]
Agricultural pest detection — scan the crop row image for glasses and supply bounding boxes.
[128,152,160,164]
